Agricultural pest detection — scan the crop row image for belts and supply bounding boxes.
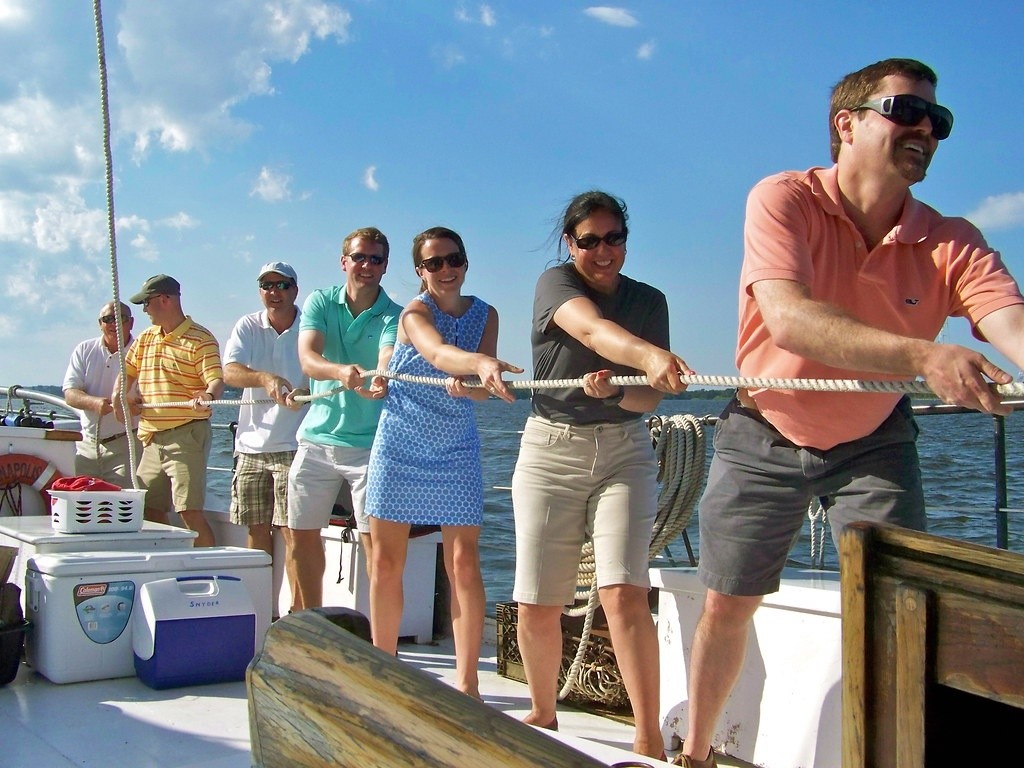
[102,429,134,444]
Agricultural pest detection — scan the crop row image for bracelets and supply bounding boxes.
[303,388,310,405]
[206,393,214,407]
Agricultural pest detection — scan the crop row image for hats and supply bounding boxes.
[256,262,297,281]
[129,274,181,305]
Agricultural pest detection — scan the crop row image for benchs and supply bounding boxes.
[648,566,843,768]
[168,492,444,644]
[0,515,199,620]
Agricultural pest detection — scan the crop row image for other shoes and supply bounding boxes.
[670,744,718,768]
[543,712,559,731]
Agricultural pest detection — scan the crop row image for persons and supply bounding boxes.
[110,273,224,547]
[286,227,403,611]
[221,261,309,557]
[511,190,695,763]
[370,226,524,703]
[669,58,1023,768]
[61,300,141,489]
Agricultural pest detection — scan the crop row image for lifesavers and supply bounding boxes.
[1,454,63,516]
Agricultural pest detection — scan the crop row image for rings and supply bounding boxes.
[491,390,496,393]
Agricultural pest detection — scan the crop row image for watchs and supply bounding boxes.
[602,386,624,408]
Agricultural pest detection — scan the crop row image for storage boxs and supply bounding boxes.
[46,489,148,534]
[26,546,273,685]
[496,600,528,684]
[132,575,258,690]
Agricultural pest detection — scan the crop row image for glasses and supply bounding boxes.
[143,294,170,307]
[344,252,386,265]
[418,250,465,273]
[570,229,627,249]
[850,94,954,140]
[99,315,131,324]
[258,280,295,290]
